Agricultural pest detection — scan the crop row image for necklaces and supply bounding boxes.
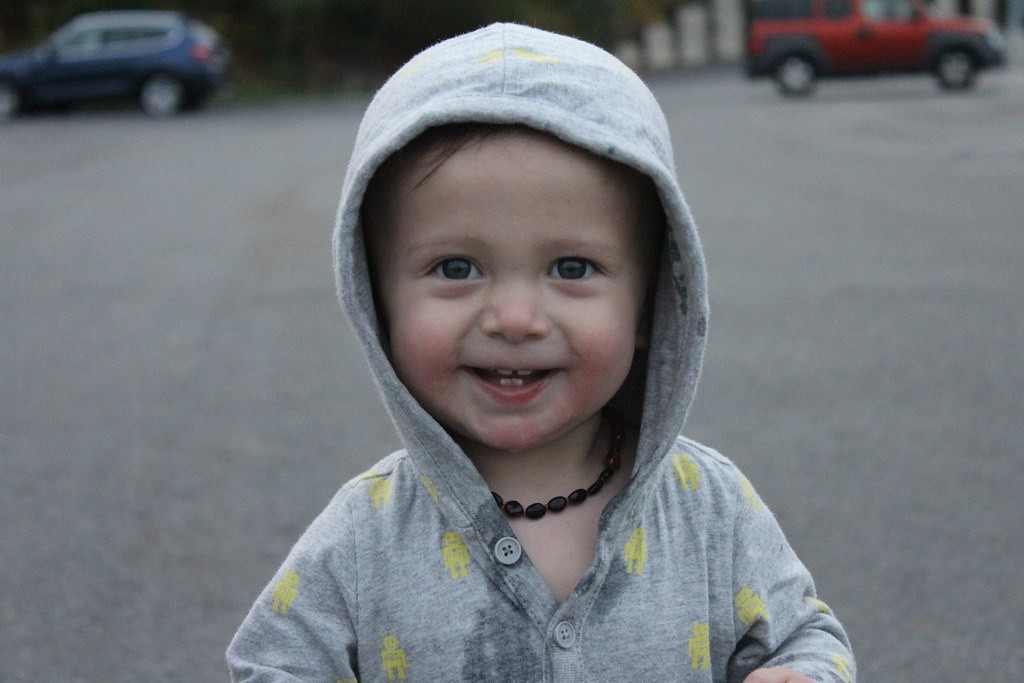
[488,401,629,518]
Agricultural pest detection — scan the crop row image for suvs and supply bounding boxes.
[0,8,239,122]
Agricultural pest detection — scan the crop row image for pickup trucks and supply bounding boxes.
[742,0,1009,99]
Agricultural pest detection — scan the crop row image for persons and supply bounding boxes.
[225,22,858,683]
